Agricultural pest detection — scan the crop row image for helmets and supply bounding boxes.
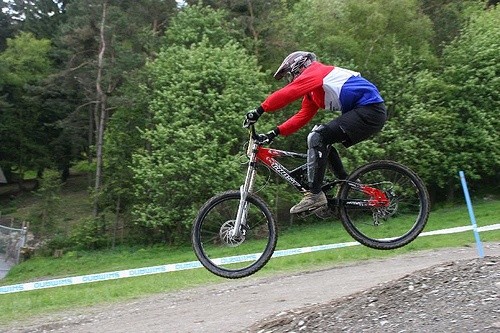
[273,51,318,82]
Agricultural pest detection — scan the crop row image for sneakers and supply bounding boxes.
[289,191,328,213]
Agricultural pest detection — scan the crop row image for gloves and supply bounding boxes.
[257,131,275,145]
[244,108,260,125]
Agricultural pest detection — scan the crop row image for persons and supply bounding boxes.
[245,50,386,214]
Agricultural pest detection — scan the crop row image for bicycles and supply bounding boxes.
[190,111,432,281]
